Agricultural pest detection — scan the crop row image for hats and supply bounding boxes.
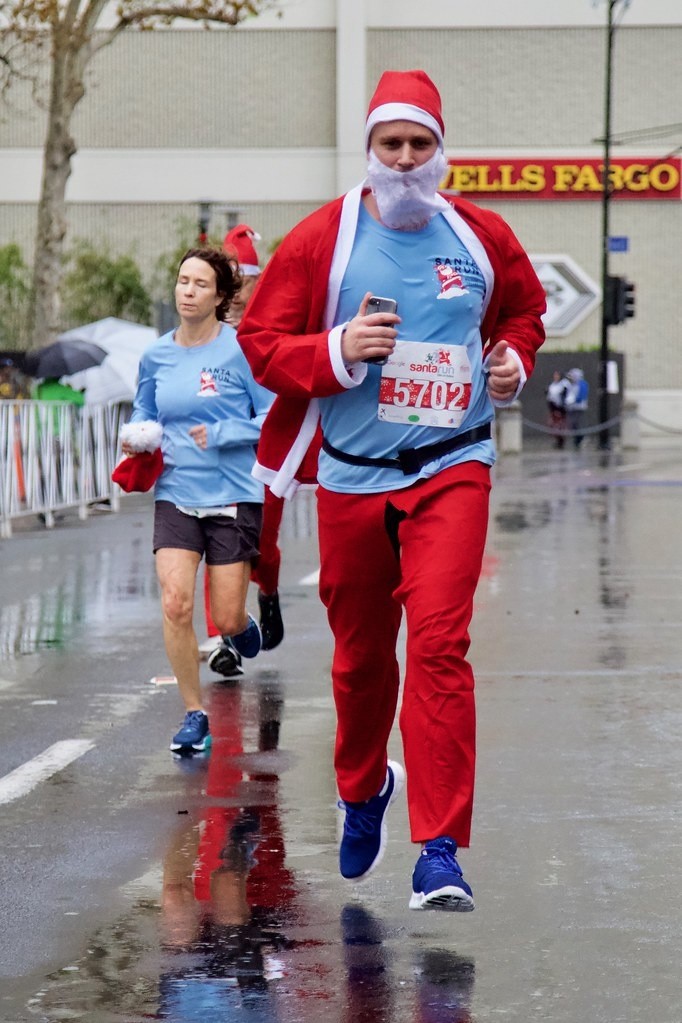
[221,225,262,278]
[113,423,165,493]
[365,69,444,153]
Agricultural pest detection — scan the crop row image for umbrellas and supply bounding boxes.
[1,313,162,413]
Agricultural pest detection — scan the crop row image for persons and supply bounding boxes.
[161,661,475,1023]
[237,68,549,911]
[129,222,284,754]
[545,370,594,448]
[0,371,112,527]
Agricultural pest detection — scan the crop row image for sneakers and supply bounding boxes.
[228,614,263,658]
[408,835,476,913]
[338,759,405,878]
[169,711,212,753]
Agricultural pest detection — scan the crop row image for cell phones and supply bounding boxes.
[361,297,397,364]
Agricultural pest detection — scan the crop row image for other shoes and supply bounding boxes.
[258,588,283,650]
[209,644,244,676]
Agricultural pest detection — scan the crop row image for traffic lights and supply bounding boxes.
[603,276,635,326]
